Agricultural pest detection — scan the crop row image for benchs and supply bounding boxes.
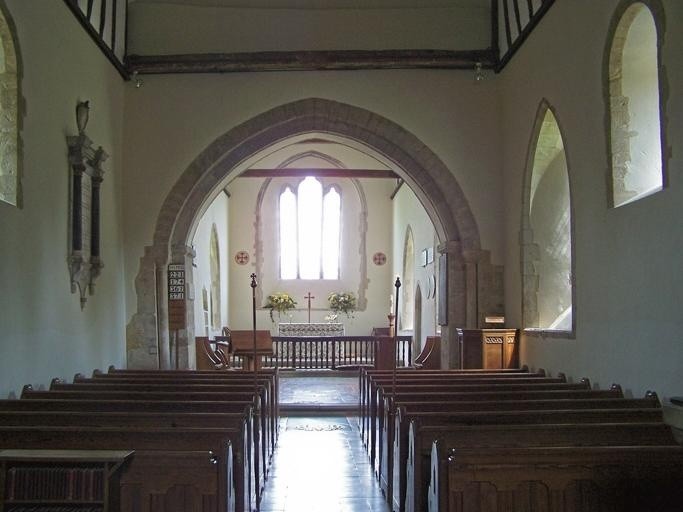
[0,365,279,511]
[355,364,682,511]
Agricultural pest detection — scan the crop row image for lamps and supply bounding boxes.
[472,63,485,82]
[131,71,143,89]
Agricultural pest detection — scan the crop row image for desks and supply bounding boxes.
[456,325,519,369]
[273,323,345,359]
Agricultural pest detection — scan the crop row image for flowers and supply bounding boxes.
[328,290,357,321]
[263,292,296,322]
